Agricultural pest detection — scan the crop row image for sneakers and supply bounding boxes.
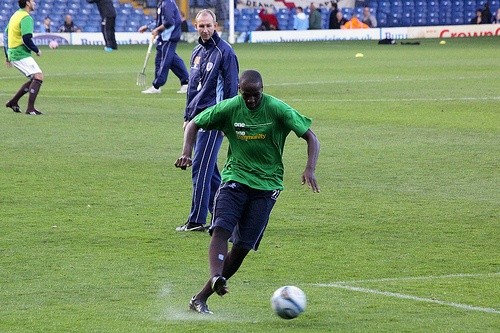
[177,84,188,93]
[25,109,43,115]
[211,274,229,296]
[6,101,22,113]
[188,296,213,315]
[175,221,204,232]
[141,86,161,94]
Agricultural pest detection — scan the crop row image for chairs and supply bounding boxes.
[0,0,500,34]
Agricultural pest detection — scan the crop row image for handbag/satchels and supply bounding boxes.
[378,38,395,44]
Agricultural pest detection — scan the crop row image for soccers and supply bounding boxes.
[270,285,307,320]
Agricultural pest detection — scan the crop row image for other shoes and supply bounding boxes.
[104,47,113,51]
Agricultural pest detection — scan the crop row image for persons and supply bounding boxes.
[329,2,377,29]
[255,7,280,31]
[138,0,190,93]
[175,9,240,232]
[57,15,82,32]
[293,6,310,30]
[471,2,500,24]
[174,70,321,315]
[3,0,44,116]
[86,0,118,51]
[307,1,322,30]
[37,16,53,32]
